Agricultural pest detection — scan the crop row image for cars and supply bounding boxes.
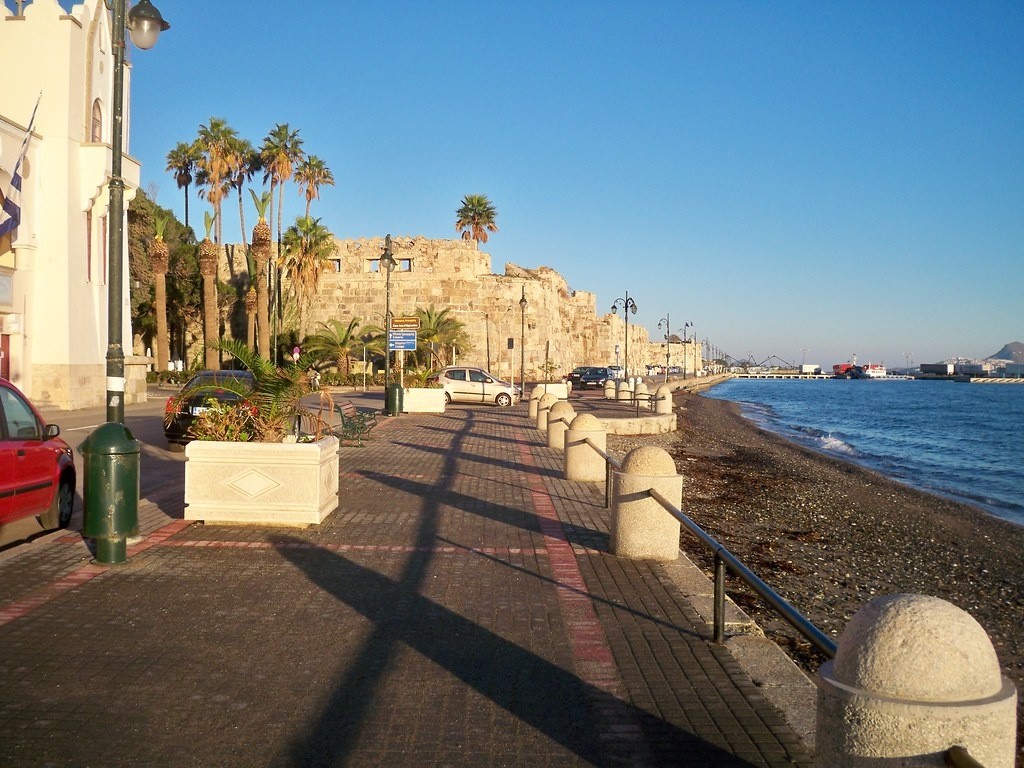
[568,366,625,390]
[424,366,522,407]
[163,370,256,446]
[0,378,77,532]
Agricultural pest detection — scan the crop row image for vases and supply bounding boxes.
[402,388,445,414]
[184,435,339,527]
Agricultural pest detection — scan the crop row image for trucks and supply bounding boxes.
[798,365,821,374]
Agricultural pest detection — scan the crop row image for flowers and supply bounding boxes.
[403,366,443,388]
[164,336,334,442]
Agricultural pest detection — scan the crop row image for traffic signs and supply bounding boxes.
[389,341,417,351]
[389,330,416,341]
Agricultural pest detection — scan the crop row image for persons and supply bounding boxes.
[307,367,317,377]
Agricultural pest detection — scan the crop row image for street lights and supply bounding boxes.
[518,282,529,399]
[105,1,170,545]
[657,313,670,383]
[677,322,699,380]
[611,291,638,384]
[378,233,398,415]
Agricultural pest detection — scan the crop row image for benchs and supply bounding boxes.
[335,400,377,446]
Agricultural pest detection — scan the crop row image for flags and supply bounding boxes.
[0,104,38,236]
